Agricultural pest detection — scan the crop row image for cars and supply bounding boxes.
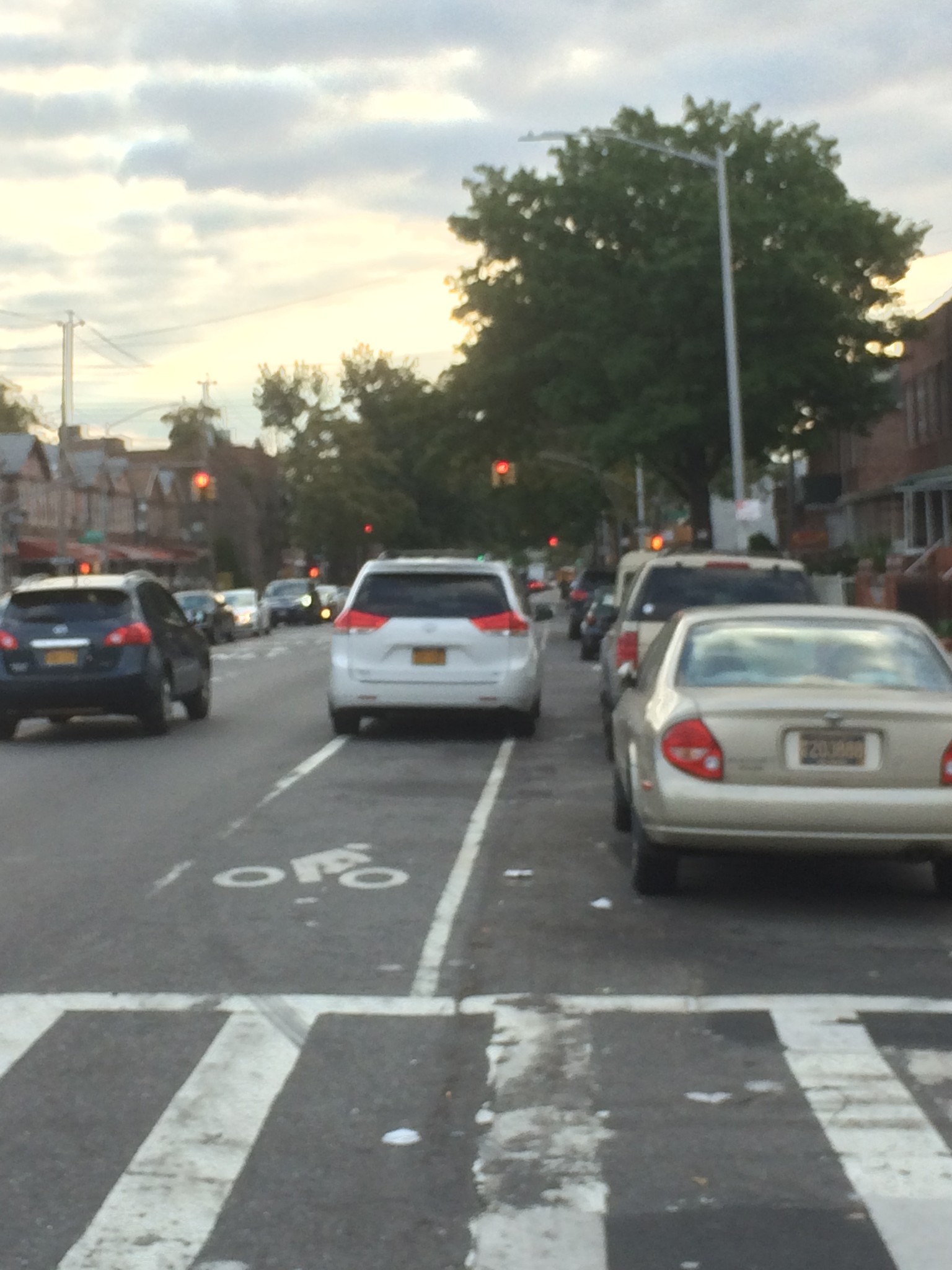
[162,575,351,644]
[607,603,951,908]
[595,548,815,711]
[321,557,551,734]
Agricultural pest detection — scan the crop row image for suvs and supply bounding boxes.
[0,567,212,728]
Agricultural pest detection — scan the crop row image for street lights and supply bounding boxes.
[525,122,752,544]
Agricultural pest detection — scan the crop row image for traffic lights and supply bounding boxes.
[182,468,216,506]
[488,457,521,489]
